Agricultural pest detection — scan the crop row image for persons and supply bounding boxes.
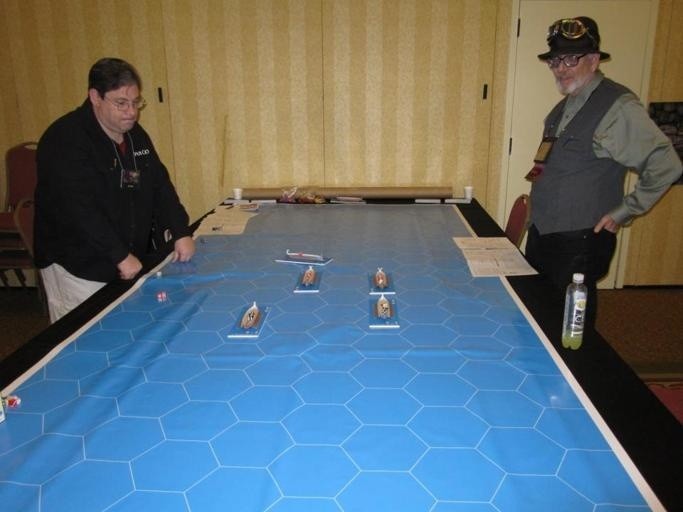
[525,16,683,327]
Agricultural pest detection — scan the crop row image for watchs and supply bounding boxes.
[33,56,197,324]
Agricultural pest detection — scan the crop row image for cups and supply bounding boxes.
[232,189,243,200]
[463,186,473,201]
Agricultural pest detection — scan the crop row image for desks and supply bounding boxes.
[1,192,683,512]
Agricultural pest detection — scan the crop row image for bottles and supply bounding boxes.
[561,273,588,350]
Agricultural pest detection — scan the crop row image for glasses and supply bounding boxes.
[546,18,598,44]
[546,55,588,68]
[106,98,146,111]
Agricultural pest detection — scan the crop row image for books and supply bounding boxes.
[453,236,540,277]
[197,211,258,235]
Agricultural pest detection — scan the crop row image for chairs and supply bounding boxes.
[1,142,39,289]
[506,194,530,248]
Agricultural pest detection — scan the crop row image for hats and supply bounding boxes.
[536,16,612,63]
